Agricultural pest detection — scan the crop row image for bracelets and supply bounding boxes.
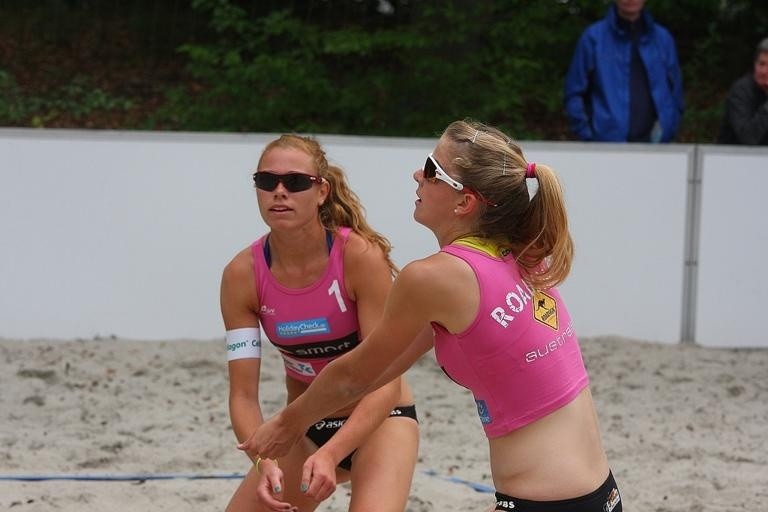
[255,456,280,476]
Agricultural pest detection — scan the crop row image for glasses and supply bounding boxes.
[252,170,327,193]
[422,151,499,209]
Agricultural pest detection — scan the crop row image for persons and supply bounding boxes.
[563,0,685,144]
[717,39,768,146]
[220,135,419,512]
[236,118,625,512]
